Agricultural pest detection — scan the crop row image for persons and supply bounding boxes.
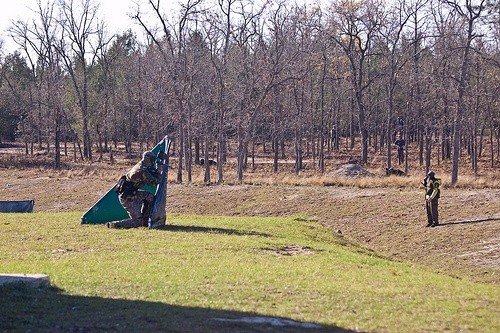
[394,134,405,163]
[421,171,441,227]
[106,151,167,228]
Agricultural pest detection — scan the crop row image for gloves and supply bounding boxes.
[156,163,163,172]
[423,176,427,185]
[428,197,432,202]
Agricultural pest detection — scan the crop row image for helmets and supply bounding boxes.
[143,150,155,165]
[428,171,434,176]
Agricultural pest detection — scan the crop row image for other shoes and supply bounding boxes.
[423,222,437,226]
[107,220,117,228]
